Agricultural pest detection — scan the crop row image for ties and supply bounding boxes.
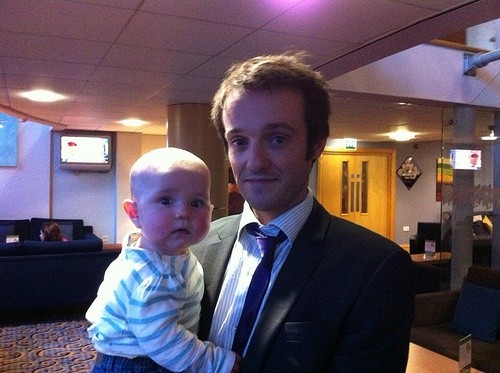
[231,221,288,355]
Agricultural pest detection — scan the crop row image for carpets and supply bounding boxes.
[0,319,98,373]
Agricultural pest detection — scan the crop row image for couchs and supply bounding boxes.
[443,210,493,266]
[0,217,122,327]
[410,222,441,254]
[410,266,500,373]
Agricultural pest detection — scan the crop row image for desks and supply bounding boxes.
[405,341,486,373]
[410,252,452,264]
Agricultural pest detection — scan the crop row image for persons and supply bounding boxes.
[84,147,242,373]
[469,150,479,169]
[39,221,68,241]
[156,48,417,373]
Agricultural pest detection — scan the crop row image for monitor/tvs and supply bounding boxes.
[60,132,113,171]
[449,149,482,170]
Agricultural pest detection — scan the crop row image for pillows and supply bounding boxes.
[448,280,500,344]
[473,215,493,236]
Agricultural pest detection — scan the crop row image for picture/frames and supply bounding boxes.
[0,112,17,166]
